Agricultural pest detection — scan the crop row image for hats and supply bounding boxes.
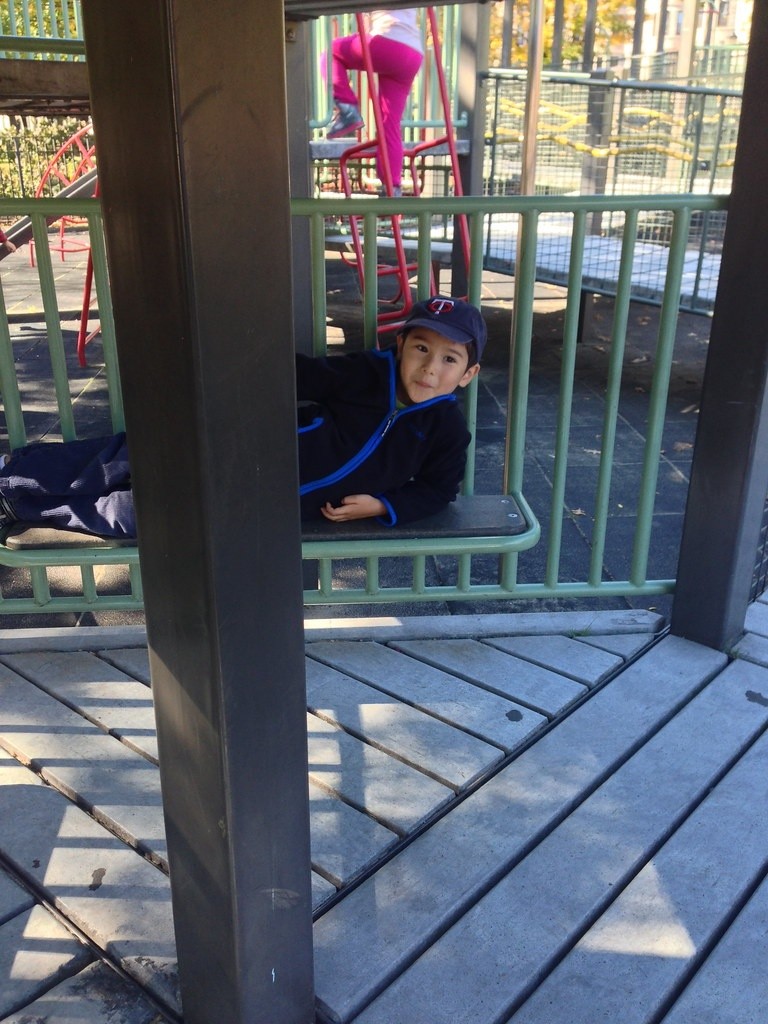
[395,294,488,363]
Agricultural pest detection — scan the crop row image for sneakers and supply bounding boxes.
[327,102,364,139]
[378,184,400,199]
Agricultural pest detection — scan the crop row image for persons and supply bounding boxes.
[0,294,487,540]
[321,8,423,198]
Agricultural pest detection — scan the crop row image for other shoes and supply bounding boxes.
[0,498,19,542]
